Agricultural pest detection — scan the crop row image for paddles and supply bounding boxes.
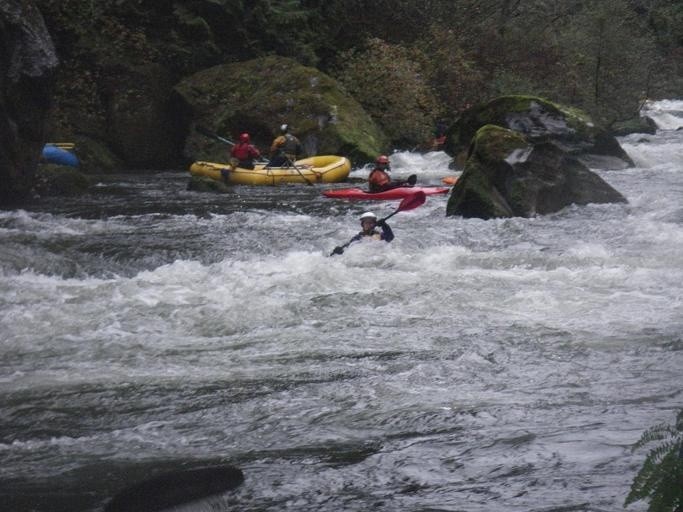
[196,125,269,162]
[329,191,426,256]
[286,158,313,186]
[382,174,417,190]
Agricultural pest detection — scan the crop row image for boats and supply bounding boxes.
[190,155,351,185]
[42,147,79,168]
[322,176,462,199]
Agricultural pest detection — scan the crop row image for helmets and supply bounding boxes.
[239,131,250,143]
[359,212,377,222]
[376,156,391,164]
[279,125,289,136]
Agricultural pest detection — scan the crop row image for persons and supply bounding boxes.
[435,132,447,145]
[230,132,260,170]
[334,212,394,254]
[263,124,305,169]
[368,155,407,191]
[416,137,438,151]
